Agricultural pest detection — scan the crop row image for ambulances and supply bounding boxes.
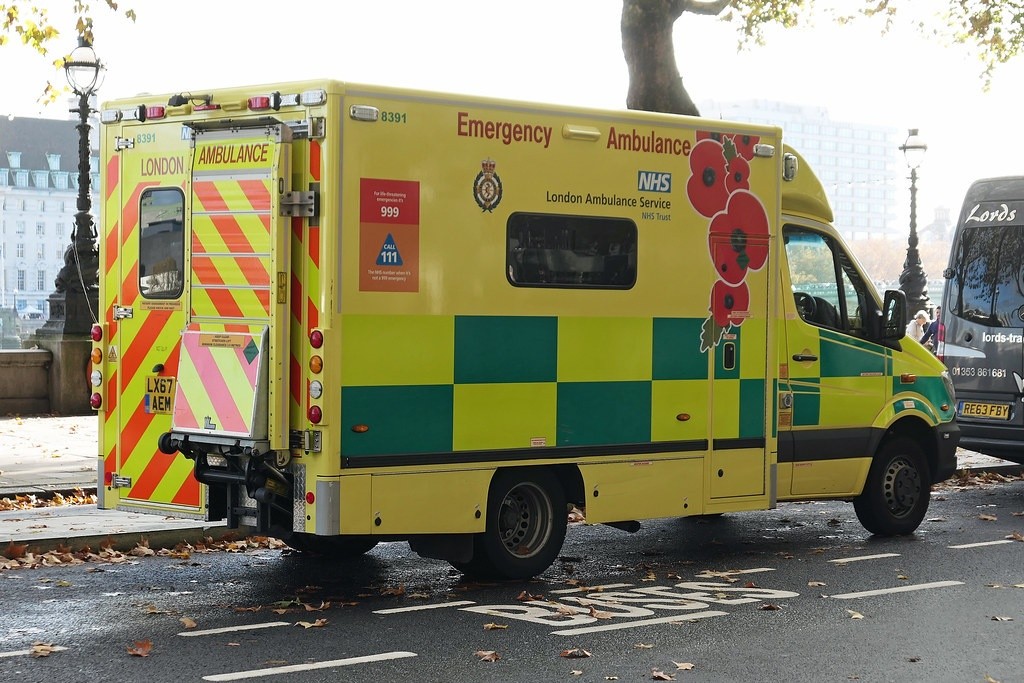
[91,77,961,584]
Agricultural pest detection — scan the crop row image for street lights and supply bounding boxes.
[898,127,928,331]
[36,45,101,340]
[13,288,18,337]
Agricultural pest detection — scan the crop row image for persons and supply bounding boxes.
[905,310,933,351]
[919,306,941,356]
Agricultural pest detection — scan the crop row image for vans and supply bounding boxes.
[936,176,1022,466]
